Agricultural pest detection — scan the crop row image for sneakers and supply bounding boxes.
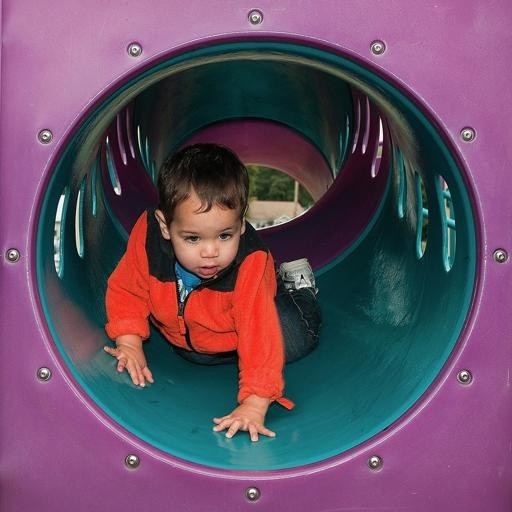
[279,258,316,294]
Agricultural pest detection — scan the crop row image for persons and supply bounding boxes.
[104,143,321,439]
